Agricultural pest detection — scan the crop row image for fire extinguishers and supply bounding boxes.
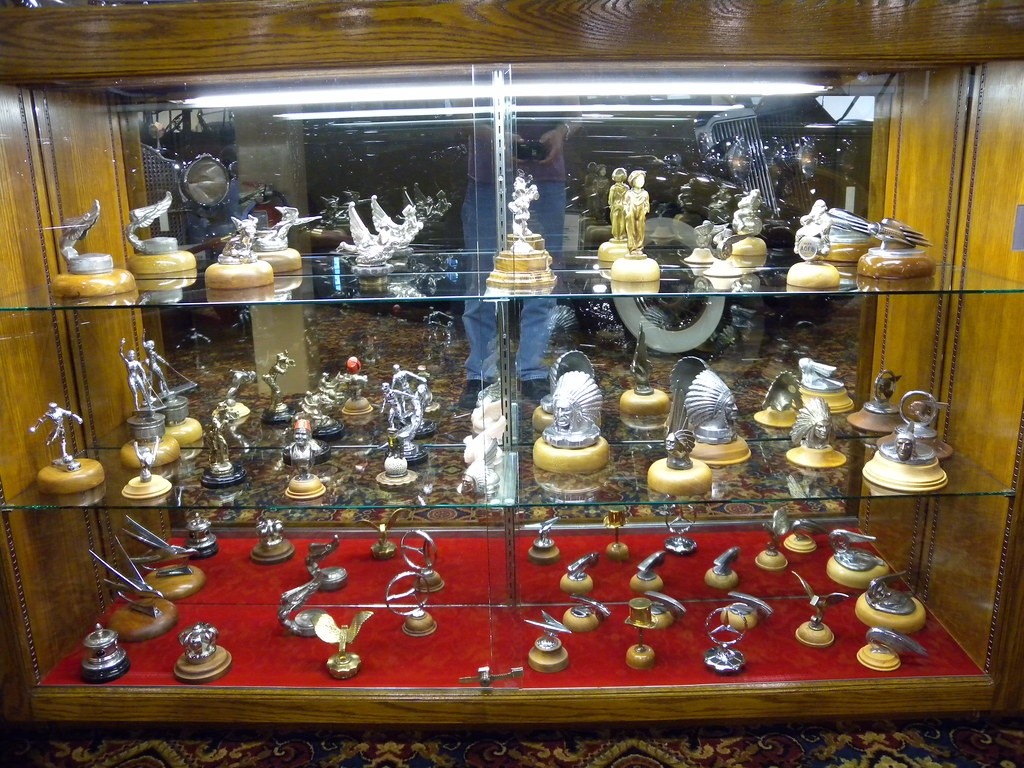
[238,181,288,230]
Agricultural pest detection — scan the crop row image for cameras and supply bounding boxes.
[507,140,545,160]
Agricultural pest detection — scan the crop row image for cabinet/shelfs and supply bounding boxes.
[0,0,1024,722]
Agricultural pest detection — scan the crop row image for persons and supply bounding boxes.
[583,161,627,241]
[541,304,579,356]
[459,461,493,494]
[684,368,739,436]
[664,430,696,458]
[289,418,323,478]
[892,420,919,461]
[793,198,833,259]
[790,398,838,449]
[550,371,604,432]
[761,370,802,412]
[457,121,585,409]
[379,381,420,445]
[119,337,154,410]
[624,168,650,255]
[291,390,337,428]
[142,325,435,414]
[29,402,84,463]
[202,401,238,463]
[675,177,762,232]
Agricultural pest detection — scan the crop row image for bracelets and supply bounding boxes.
[564,122,570,142]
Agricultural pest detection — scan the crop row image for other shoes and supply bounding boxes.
[459,379,491,409]
[519,378,550,403]
